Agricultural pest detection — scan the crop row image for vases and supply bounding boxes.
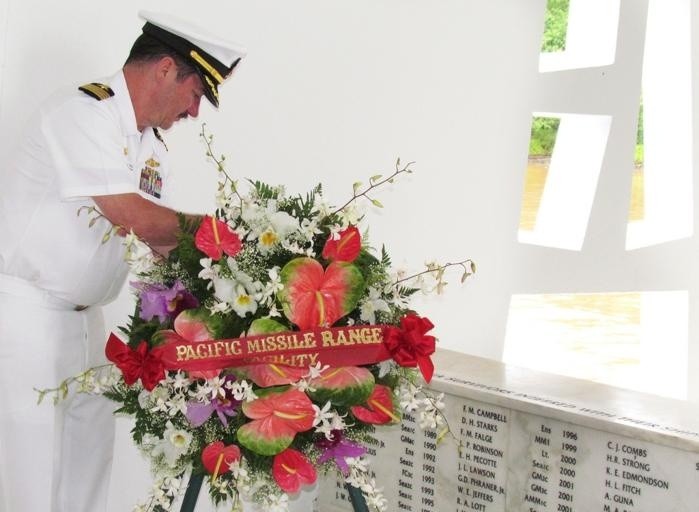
[227,467,318,512]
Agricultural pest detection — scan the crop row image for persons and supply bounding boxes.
[1,11,251,510]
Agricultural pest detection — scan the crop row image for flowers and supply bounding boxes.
[33,122,476,512]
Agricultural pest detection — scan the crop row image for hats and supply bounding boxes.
[139,10,246,110]
